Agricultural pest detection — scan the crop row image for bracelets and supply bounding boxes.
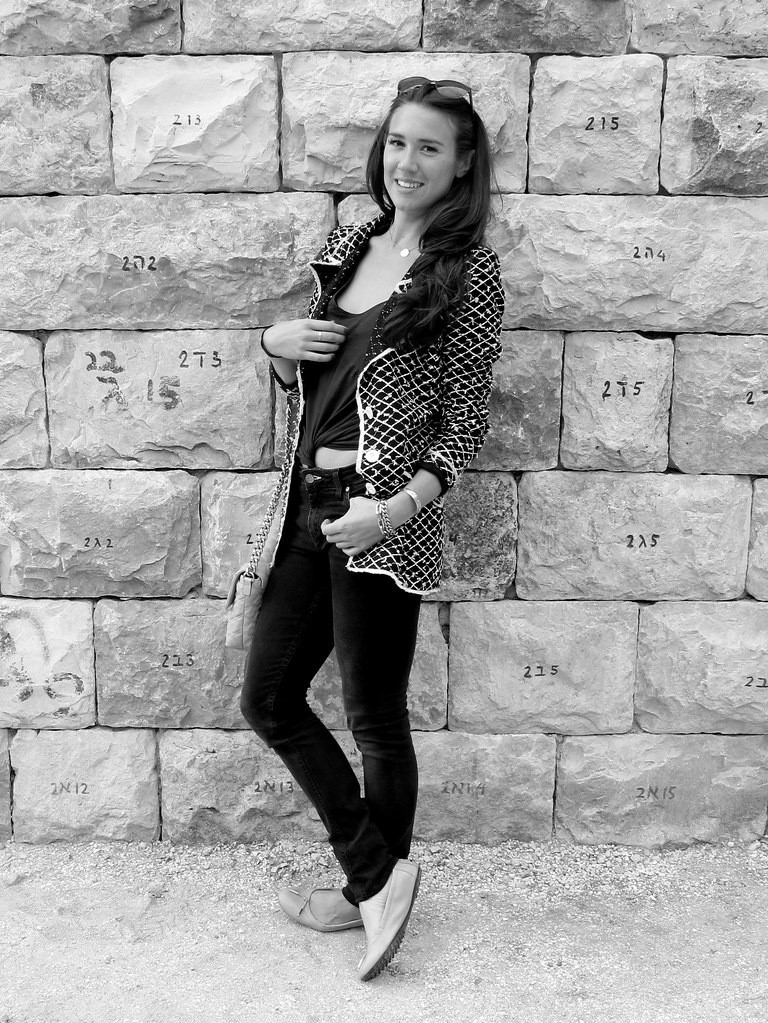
[401,488,421,514]
[261,325,282,358]
[376,499,395,538]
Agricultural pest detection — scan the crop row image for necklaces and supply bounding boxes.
[389,231,418,257]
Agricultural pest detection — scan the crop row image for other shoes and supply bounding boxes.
[279,885,363,932]
[360,859,422,980]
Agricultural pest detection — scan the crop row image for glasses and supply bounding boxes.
[396,76,475,112]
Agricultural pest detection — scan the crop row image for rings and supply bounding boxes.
[319,332,321,340]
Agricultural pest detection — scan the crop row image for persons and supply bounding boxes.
[238,77,506,980]
